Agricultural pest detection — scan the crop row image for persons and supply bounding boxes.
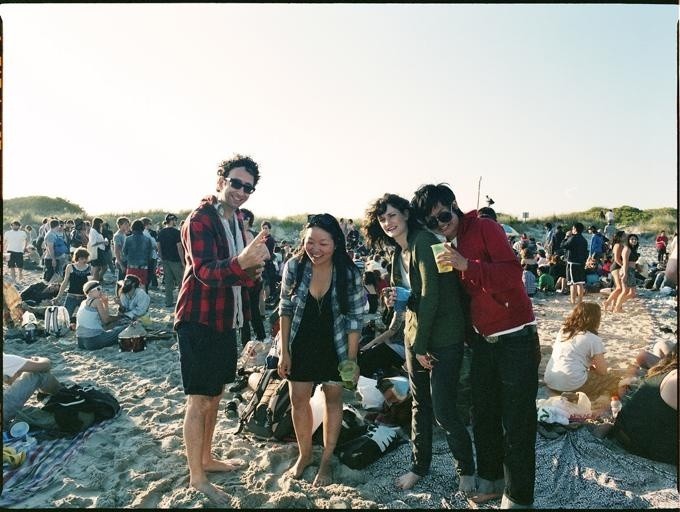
[412,184,541,510]
[363,195,476,493]
[277,213,364,488]
[174,156,269,508]
[614,350,679,465]
[476,207,677,369]
[485,192,494,205]
[1,353,66,424]
[234,208,406,370]
[545,300,617,403]
[2,213,186,350]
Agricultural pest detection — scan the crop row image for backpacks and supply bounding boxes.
[238,368,293,438]
[45,306,69,337]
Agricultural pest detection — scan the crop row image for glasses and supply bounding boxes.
[226,177,254,194]
[308,215,332,226]
[427,204,453,228]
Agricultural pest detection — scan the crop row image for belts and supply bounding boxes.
[483,324,537,343]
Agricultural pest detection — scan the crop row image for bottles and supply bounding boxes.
[25,314,36,343]
[610,395,624,420]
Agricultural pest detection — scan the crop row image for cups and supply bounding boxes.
[393,286,412,314]
[338,358,357,390]
[429,241,455,274]
